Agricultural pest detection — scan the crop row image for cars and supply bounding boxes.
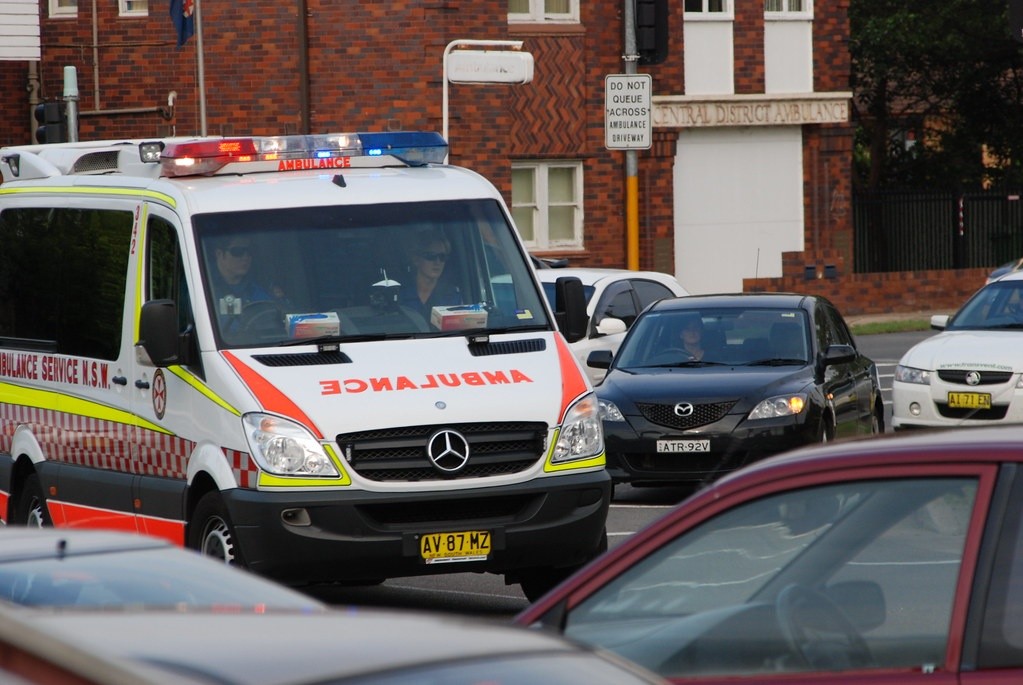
[0,522,329,610]
[507,419,1023,685]
[1,588,671,685]
[487,262,714,389]
[583,288,888,488]
[888,255,1022,431]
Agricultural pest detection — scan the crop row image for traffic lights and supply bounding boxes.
[33,99,67,144]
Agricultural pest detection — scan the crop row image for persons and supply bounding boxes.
[183,235,269,323]
[679,318,722,362]
[398,229,463,318]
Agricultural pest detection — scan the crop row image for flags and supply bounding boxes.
[170,0,194,48]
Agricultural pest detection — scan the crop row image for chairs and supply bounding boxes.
[750,323,804,365]
[702,328,727,363]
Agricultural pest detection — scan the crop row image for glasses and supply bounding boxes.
[417,250,449,261]
[219,246,258,257]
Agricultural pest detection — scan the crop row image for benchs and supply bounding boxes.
[725,337,767,365]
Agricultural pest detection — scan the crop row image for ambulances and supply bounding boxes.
[0,125,613,607]
